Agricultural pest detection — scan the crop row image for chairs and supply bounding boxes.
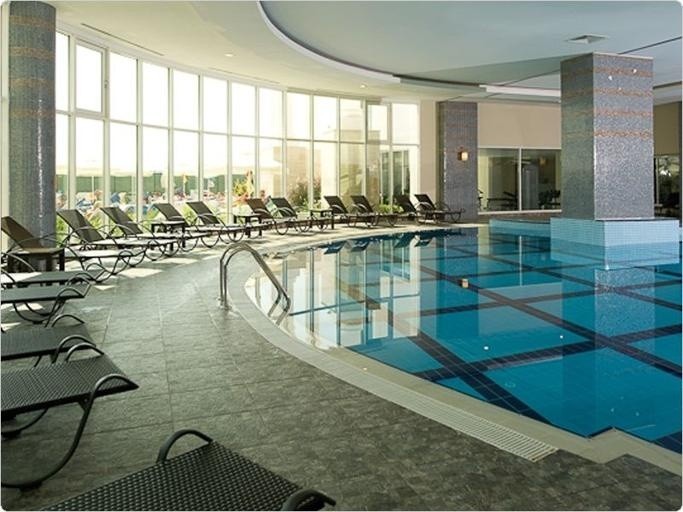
[154,203,248,247]
[3,274,91,323]
[654,192,680,215]
[396,194,437,223]
[3,312,96,363]
[326,196,380,229]
[2,217,146,286]
[2,253,105,285]
[57,209,179,267]
[101,207,212,255]
[53,427,335,510]
[0,343,140,485]
[245,198,313,234]
[350,194,397,226]
[416,195,463,223]
[273,197,332,230]
[189,201,271,240]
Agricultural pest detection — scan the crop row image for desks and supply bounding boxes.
[232,215,262,237]
[150,221,186,250]
[309,209,332,229]
[11,246,67,286]
